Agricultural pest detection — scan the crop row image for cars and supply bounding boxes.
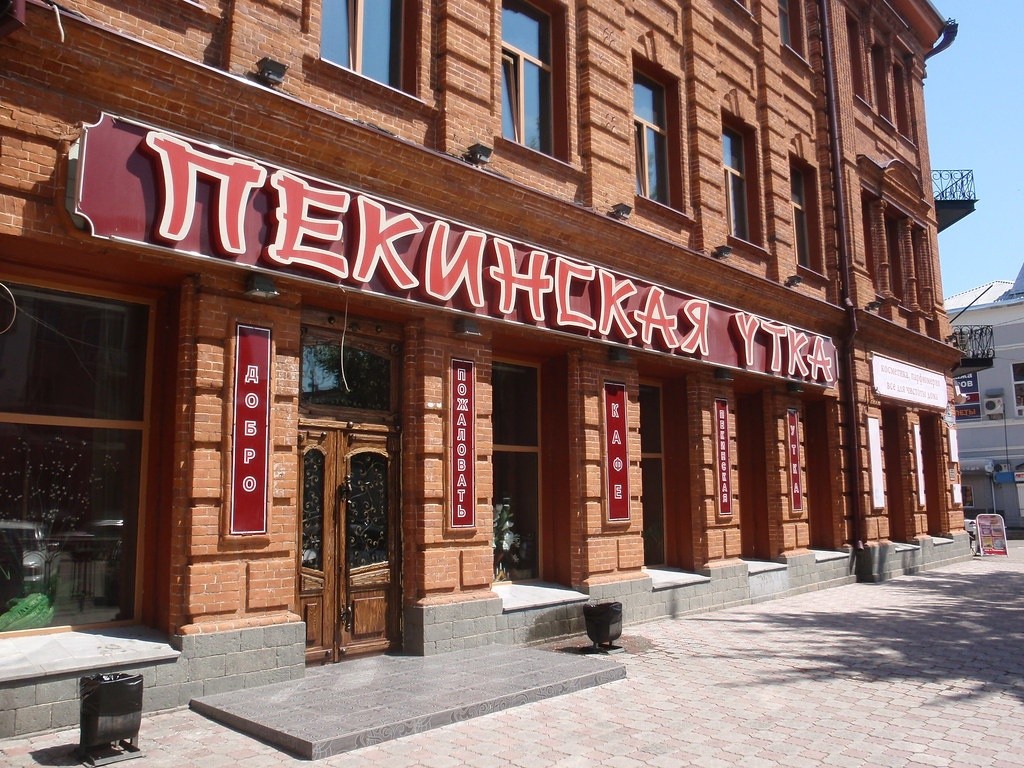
[0,520,61,602]
[86,520,123,594]
[964,519,977,534]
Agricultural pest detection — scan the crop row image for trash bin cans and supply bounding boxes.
[580,601,625,656]
[68,671,147,768]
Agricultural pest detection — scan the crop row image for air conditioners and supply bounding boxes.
[993,464,1012,478]
[983,398,1004,415]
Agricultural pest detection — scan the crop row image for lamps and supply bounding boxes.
[607,202,632,220]
[784,274,803,288]
[711,245,732,260]
[864,301,882,312]
[255,57,290,89]
[944,335,957,344]
[462,144,494,166]
